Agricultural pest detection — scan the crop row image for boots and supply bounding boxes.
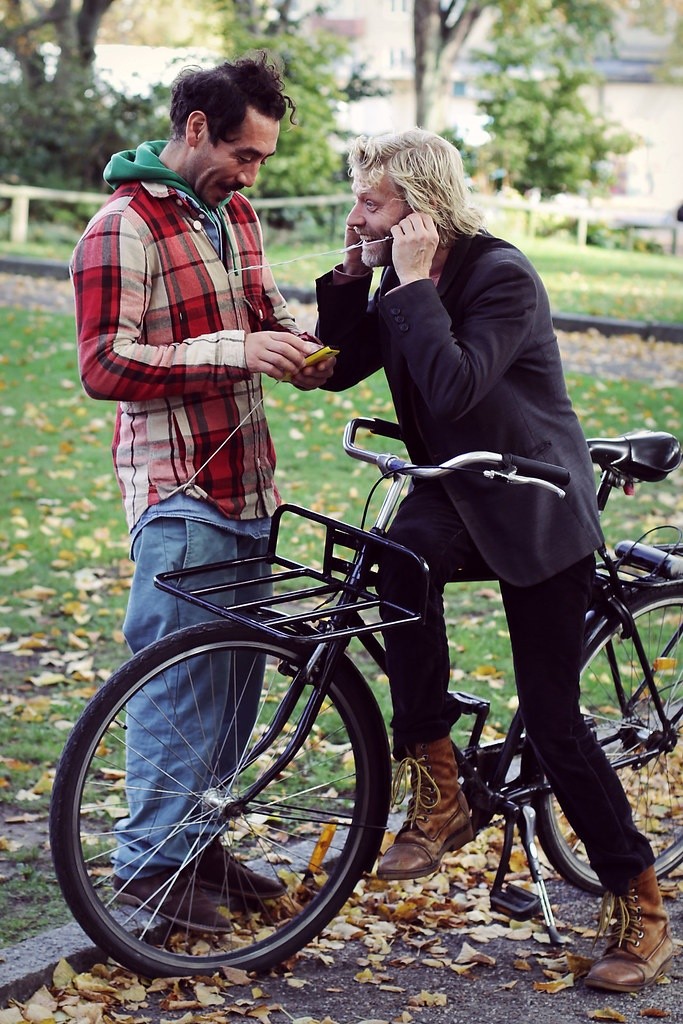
[375,734,474,880]
[582,865,674,993]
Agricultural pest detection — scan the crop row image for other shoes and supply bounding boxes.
[190,836,287,900]
[111,865,233,934]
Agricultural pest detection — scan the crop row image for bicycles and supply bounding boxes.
[49,414,683,988]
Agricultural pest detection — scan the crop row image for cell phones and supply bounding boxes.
[277,346,340,382]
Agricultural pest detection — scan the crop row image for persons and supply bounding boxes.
[66,59,337,935]
[314,129,673,993]
